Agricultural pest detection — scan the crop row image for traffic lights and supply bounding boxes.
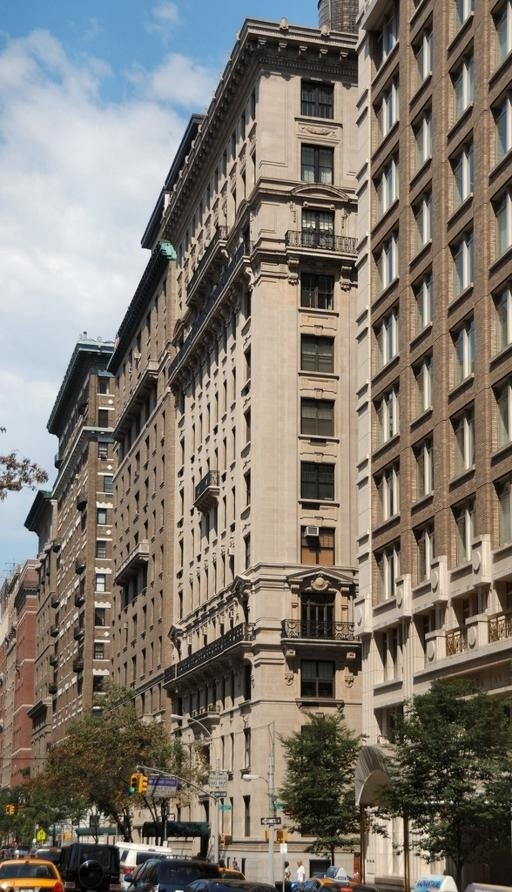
[129,772,148,793]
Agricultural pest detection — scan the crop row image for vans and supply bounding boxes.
[114,840,175,892]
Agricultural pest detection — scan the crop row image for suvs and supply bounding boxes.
[51,840,121,892]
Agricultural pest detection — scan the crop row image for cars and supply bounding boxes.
[123,857,380,892]
[0,858,62,892]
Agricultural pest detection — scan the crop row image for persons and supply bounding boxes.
[296,859,305,884]
[217,859,225,867]
[283,861,291,881]
[232,860,240,873]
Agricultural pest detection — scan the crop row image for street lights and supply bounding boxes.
[241,754,277,887]
[135,713,221,862]
